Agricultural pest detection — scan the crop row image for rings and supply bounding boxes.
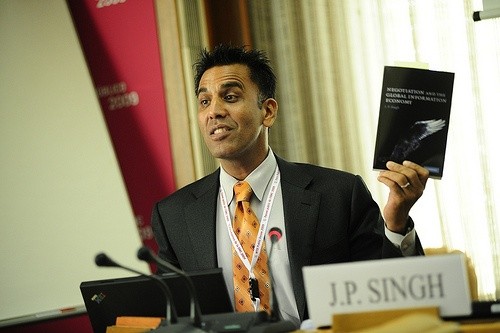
[401,183,410,188]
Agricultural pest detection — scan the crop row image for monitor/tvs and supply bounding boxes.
[79,267,236,333]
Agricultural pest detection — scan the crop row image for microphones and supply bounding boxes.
[136,247,206,329]
[94,253,180,324]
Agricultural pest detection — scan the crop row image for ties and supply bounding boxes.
[233,181,271,311]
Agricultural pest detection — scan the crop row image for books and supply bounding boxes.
[373,66,455,179]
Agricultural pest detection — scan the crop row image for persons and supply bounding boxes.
[152,41,429,328]
